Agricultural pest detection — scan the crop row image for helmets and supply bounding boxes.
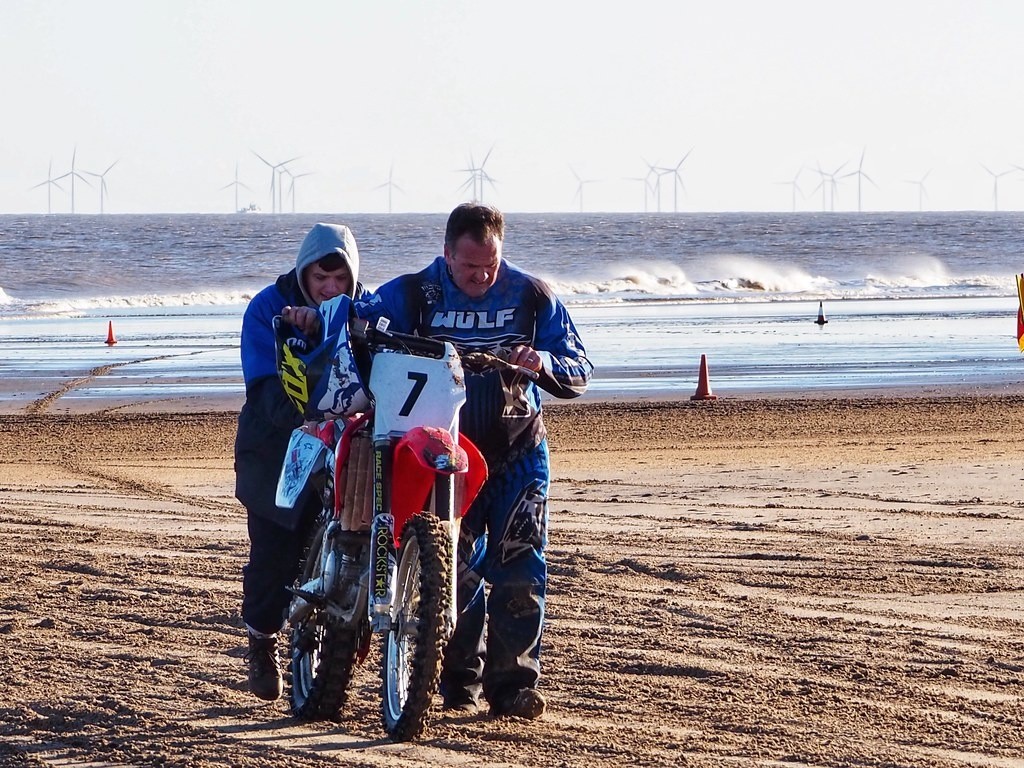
[272,293,371,422]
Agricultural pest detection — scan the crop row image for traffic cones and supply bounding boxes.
[690,353,716,400]
[104,321,117,344]
[813,300,828,323]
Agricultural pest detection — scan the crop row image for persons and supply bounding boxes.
[234,203,596,719]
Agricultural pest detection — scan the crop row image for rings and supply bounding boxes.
[528,358,533,362]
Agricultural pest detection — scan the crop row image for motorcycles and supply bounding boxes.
[280,313,540,743]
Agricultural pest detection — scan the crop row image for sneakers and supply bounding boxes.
[445,694,478,714]
[490,688,544,719]
[244,630,283,700]
